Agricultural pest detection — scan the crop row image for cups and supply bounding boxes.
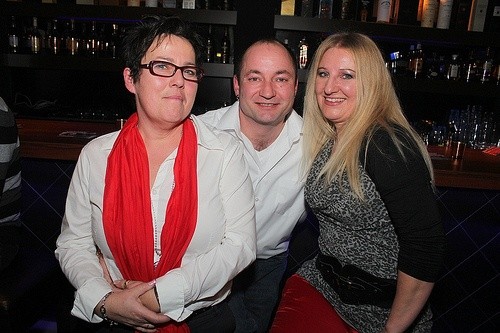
[449,105,496,150]
[451,142,467,160]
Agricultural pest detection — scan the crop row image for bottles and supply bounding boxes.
[8,14,125,59]
[391,43,460,85]
[221,42,228,63]
[42,0,231,10]
[204,40,214,63]
[481,47,493,84]
[281,0,500,31]
[285,38,309,69]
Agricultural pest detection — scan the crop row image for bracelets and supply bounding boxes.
[100,292,118,326]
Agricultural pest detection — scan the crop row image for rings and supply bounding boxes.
[124,280,130,289]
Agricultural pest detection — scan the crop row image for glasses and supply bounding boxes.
[134,60,205,82]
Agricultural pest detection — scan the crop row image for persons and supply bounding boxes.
[269,32,441,333]
[184,37,332,333]
[55,15,257,333]
[0,95,24,224]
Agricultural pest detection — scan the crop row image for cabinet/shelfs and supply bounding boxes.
[0,0,238,78]
[271,14,500,97]
